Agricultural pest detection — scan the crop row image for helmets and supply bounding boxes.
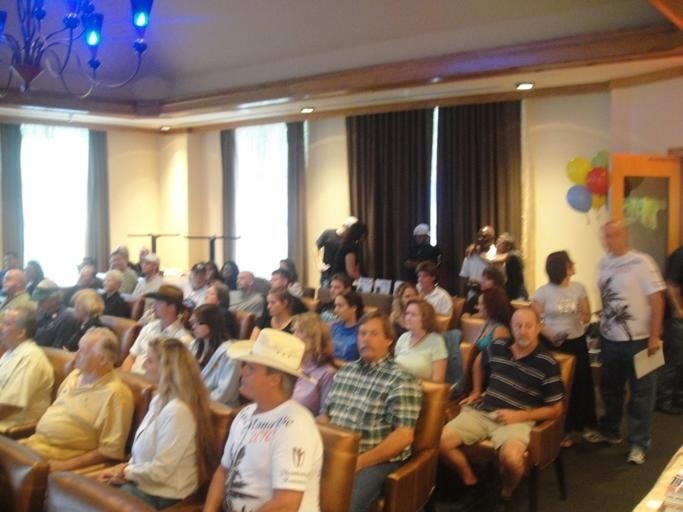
[413,224,431,238]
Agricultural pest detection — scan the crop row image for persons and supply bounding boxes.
[332,222,368,284]
[62,288,113,352]
[122,285,194,376]
[466,233,529,302]
[278,260,304,296]
[662,243,683,412]
[403,224,443,283]
[324,292,365,359]
[100,338,216,509]
[460,267,507,324]
[23,261,45,300]
[316,272,360,330]
[131,255,165,299]
[203,281,237,337]
[389,284,420,346]
[202,260,229,292]
[131,247,149,277]
[315,312,424,512]
[0,269,38,327]
[177,264,217,307]
[393,300,448,383]
[530,250,604,449]
[0,253,23,307]
[228,271,264,321]
[596,219,667,463]
[75,258,104,306]
[99,269,131,317]
[457,225,507,307]
[220,261,238,289]
[439,307,565,512]
[102,250,136,294]
[450,288,512,417]
[250,287,303,342]
[314,216,359,290]
[200,329,324,512]
[14,327,134,471]
[1,306,55,434]
[32,279,79,347]
[263,268,309,317]
[409,261,453,317]
[288,312,338,419]
[187,304,240,407]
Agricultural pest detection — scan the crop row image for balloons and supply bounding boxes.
[590,150,610,170]
[567,187,593,213]
[590,195,607,210]
[566,156,591,183]
[586,169,610,196]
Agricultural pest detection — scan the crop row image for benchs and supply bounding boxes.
[317,289,393,314]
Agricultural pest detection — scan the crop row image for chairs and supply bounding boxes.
[10,347,77,440]
[447,352,574,512]
[458,342,472,374]
[310,422,361,512]
[436,316,452,333]
[253,279,271,298]
[329,360,452,512]
[0,432,50,512]
[125,297,145,320]
[232,312,255,340]
[453,297,466,330]
[97,316,142,369]
[299,296,320,313]
[48,471,154,512]
[9,372,154,475]
[84,391,232,512]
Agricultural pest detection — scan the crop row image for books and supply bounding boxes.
[633,342,665,379]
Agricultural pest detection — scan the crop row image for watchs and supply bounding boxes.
[118,464,129,482]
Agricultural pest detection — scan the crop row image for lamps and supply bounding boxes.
[0,0,154,99]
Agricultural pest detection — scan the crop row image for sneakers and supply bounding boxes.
[452,483,486,509]
[627,445,647,464]
[492,490,511,512]
[584,431,599,441]
[561,433,574,447]
[590,432,622,445]
[656,402,681,414]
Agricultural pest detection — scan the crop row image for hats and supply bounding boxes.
[141,254,160,265]
[31,281,59,302]
[143,286,187,308]
[190,263,207,272]
[227,328,319,385]
[344,216,358,227]
